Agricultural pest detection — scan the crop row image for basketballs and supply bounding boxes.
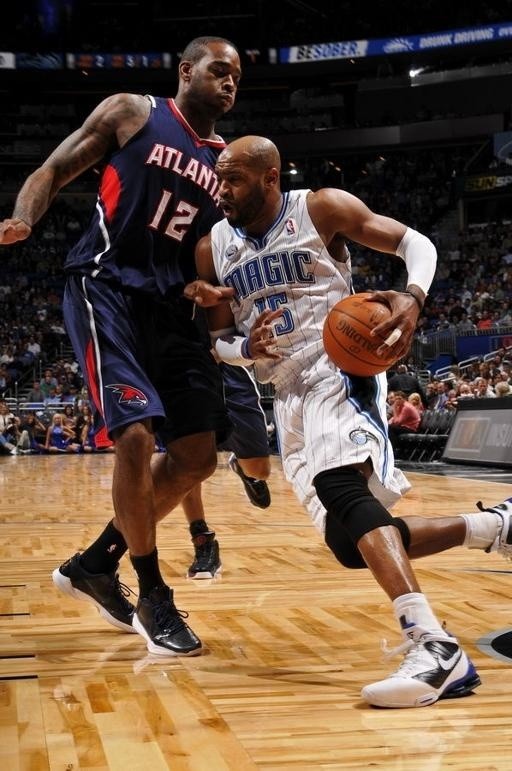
[323,293,402,375]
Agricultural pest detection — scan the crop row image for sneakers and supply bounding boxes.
[50,551,139,633]
[227,450,272,510]
[128,585,203,658]
[486,490,512,565]
[358,631,483,708]
[185,530,223,579]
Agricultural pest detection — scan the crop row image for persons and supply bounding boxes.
[190,134,512,707]
[181,364,273,582]
[0,34,242,658]
